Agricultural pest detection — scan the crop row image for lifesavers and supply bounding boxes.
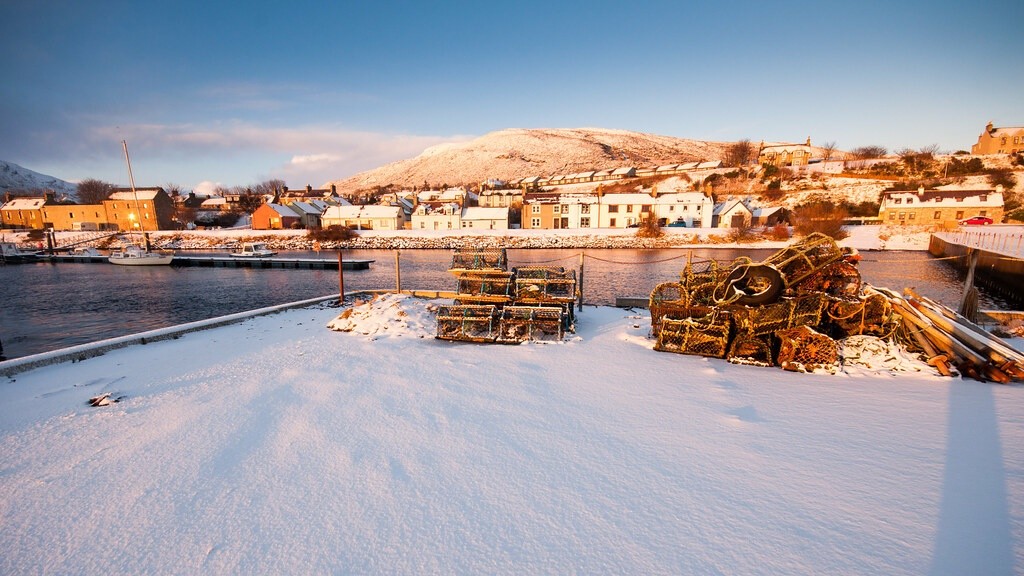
[724,264,783,306]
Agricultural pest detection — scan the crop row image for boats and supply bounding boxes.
[108,244,175,265]
[68,247,103,256]
[229,242,277,258]
